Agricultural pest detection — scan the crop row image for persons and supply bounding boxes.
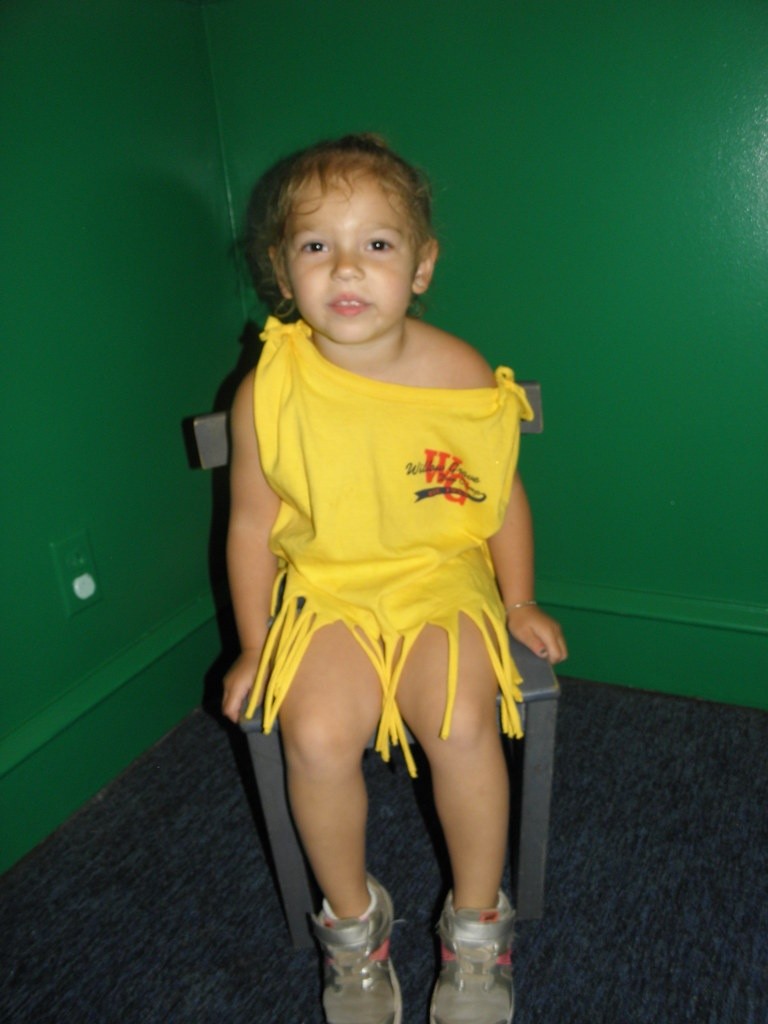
[220,130,570,1024]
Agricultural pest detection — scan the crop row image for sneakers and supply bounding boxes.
[430,886,517,1024]
[310,872,403,1024]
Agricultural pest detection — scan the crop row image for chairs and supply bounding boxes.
[193,383,562,950]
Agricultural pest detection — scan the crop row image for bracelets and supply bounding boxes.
[506,600,537,614]
